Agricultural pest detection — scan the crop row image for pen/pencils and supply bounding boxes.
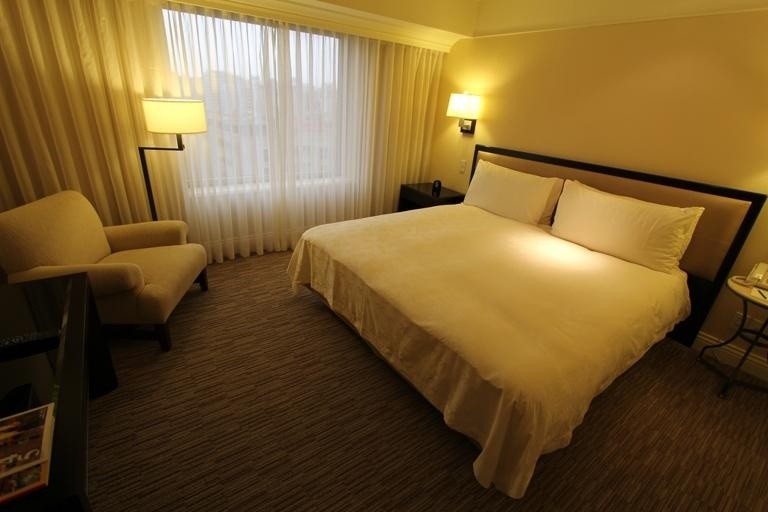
[758,290,767,299]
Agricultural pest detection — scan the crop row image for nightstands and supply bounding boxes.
[397,182,464,212]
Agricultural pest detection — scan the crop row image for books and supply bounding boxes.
[0,416,57,502]
[0,401,54,478]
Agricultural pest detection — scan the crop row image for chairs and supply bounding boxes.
[0,191,207,351]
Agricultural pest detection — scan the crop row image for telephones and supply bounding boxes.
[745,262,768,290]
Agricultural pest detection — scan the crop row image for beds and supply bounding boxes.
[285,144,768,500]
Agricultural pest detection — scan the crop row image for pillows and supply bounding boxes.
[465,160,556,226]
[548,179,697,273]
[574,179,705,270]
[485,161,564,226]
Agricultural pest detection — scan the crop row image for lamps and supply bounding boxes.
[137,97,207,222]
[446,94,480,133]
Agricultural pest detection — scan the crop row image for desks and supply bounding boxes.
[694,277,768,398]
[0,272,119,512]
